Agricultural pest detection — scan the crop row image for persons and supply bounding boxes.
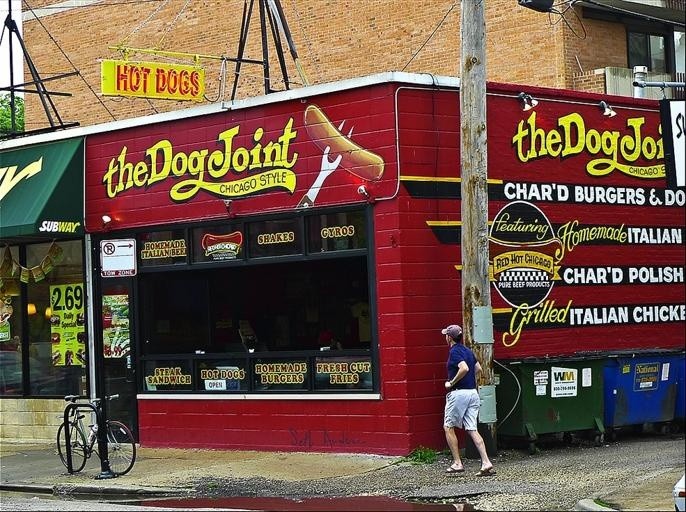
[439,324,496,476]
[16,339,38,372]
[266,314,306,361]
[351,299,370,349]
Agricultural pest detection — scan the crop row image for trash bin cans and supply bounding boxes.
[496,348,686,454]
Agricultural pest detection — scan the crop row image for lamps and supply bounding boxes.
[358,185,370,198]
[518,92,539,113]
[599,101,617,118]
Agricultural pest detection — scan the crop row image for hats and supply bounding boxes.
[441,324,463,338]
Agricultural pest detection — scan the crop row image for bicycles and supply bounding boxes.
[57,394,136,476]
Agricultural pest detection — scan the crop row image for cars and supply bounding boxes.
[0,342,82,395]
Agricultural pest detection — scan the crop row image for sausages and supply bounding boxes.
[304,105,385,182]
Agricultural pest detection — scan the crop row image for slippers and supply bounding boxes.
[445,466,465,473]
[476,468,496,476]
